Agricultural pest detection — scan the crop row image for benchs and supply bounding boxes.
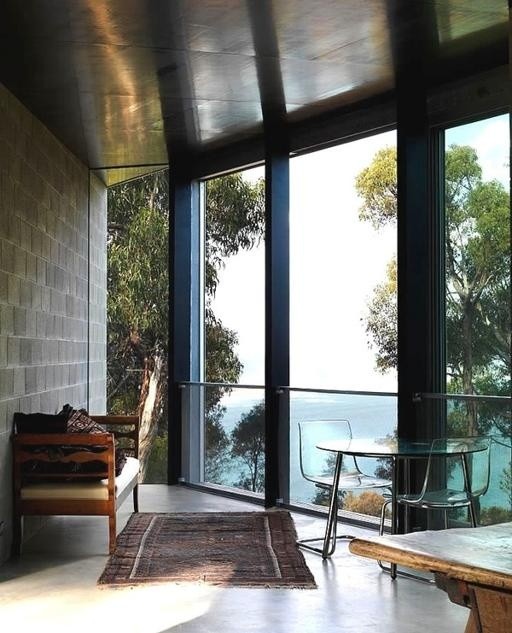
[11,413,144,560]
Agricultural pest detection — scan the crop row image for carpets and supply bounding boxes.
[97,510,320,591]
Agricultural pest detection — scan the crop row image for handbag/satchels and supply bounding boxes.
[12,404,126,482]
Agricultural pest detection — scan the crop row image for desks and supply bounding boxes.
[349,521,512,633]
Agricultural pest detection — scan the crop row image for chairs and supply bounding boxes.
[297,419,492,588]
